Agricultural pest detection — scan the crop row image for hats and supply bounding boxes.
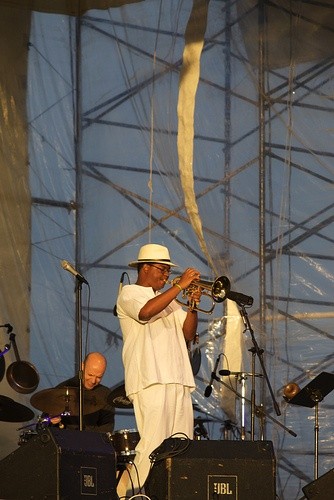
[127,243,177,269]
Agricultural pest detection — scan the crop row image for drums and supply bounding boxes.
[18,430,39,446]
[113,428,140,456]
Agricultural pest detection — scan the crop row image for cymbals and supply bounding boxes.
[29,387,107,416]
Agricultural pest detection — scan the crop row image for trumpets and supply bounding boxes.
[162,276,232,315]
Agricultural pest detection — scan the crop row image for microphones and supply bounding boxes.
[226,291,254,305]
[204,357,220,398]
[219,370,262,377]
[60,259,88,285]
[113,274,124,316]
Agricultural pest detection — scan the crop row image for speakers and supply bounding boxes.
[143,439,276,500]
[0,426,117,500]
[302,468,334,500]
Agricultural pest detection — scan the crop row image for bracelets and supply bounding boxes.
[175,284,182,291]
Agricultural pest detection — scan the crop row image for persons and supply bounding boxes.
[36,352,115,433]
[116,243,203,500]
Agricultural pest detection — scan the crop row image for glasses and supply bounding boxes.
[150,265,172,272]
